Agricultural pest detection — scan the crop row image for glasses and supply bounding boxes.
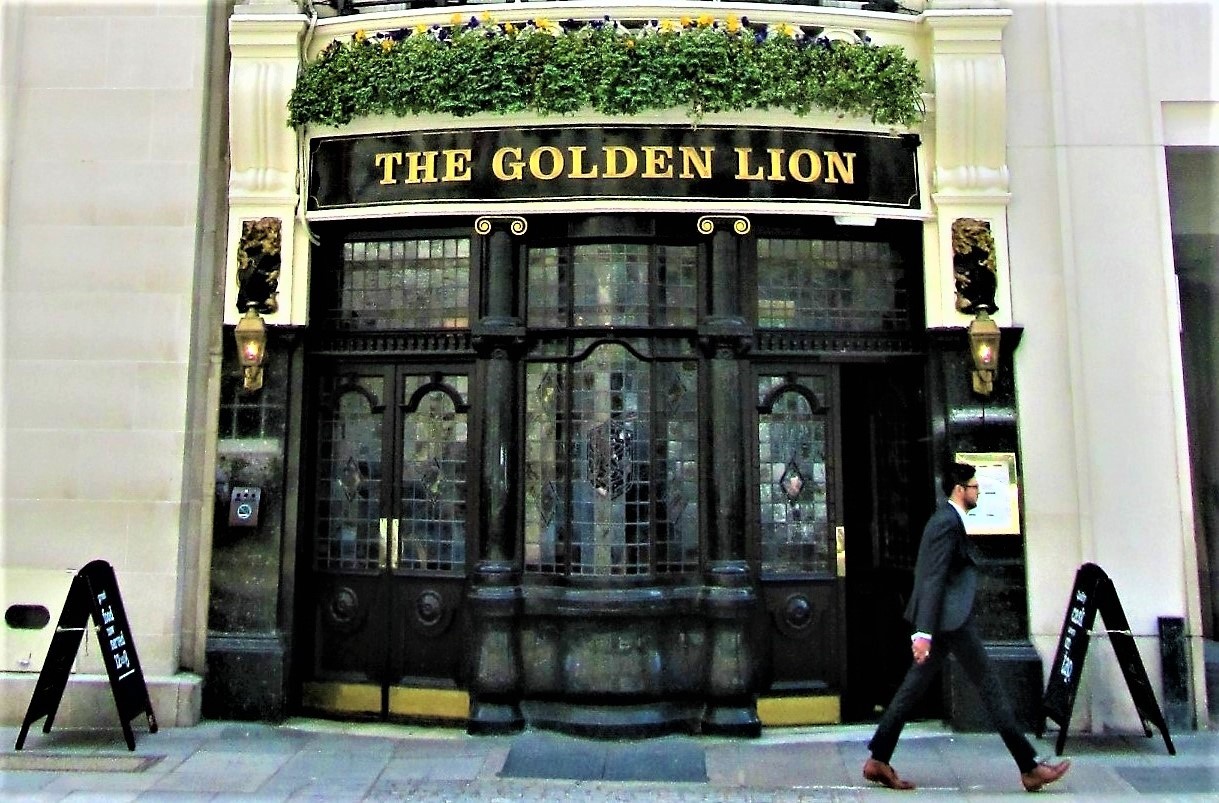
[959,483,980,491]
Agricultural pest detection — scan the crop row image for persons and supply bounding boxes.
[861,463,1073,792]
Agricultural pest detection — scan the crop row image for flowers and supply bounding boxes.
[290,12,927,130]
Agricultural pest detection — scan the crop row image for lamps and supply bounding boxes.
[233,301,267,389]
[966,305,1000,396]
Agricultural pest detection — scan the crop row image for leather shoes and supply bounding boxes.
[862,758,915,790]
[1021,755,1070,792]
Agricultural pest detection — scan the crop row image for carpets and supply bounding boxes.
[496,728,706,785]
